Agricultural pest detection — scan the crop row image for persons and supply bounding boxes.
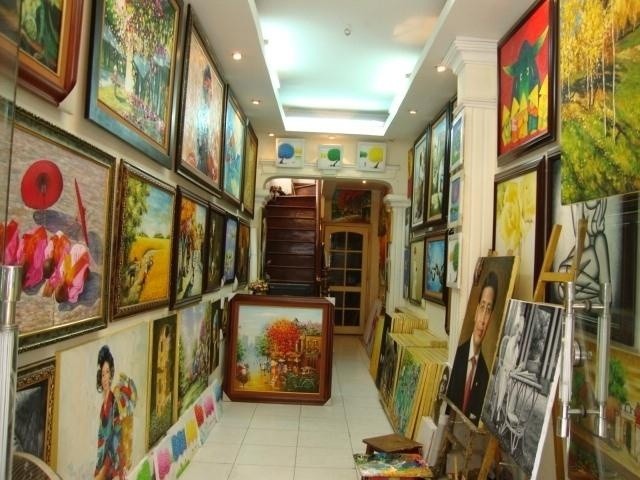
[447,270,498,425]
[92,346,137,479]
[492,316,525,422]
[430,365,450,425]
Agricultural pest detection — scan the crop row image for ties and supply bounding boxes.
[463,357,476,413]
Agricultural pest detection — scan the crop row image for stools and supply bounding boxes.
[363,433,423,455]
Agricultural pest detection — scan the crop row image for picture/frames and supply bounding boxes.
[362,299,449,441]
[545,144,639,346]
[496,0,559,167]
[222,292,336,406]
[492,156,546,304]
[316,143,344,170]
[355,141,387,172]
[275,137,304,169]
[403,92,466,309]
